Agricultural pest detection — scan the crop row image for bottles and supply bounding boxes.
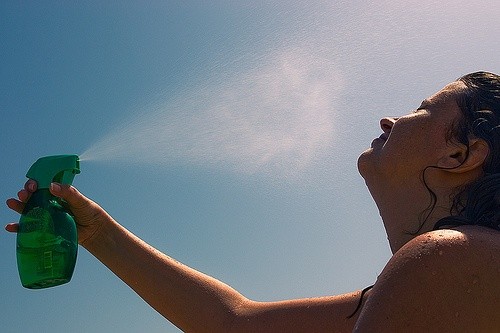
[15,153,81,289]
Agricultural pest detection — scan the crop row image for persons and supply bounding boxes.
[3,67,500,333]
